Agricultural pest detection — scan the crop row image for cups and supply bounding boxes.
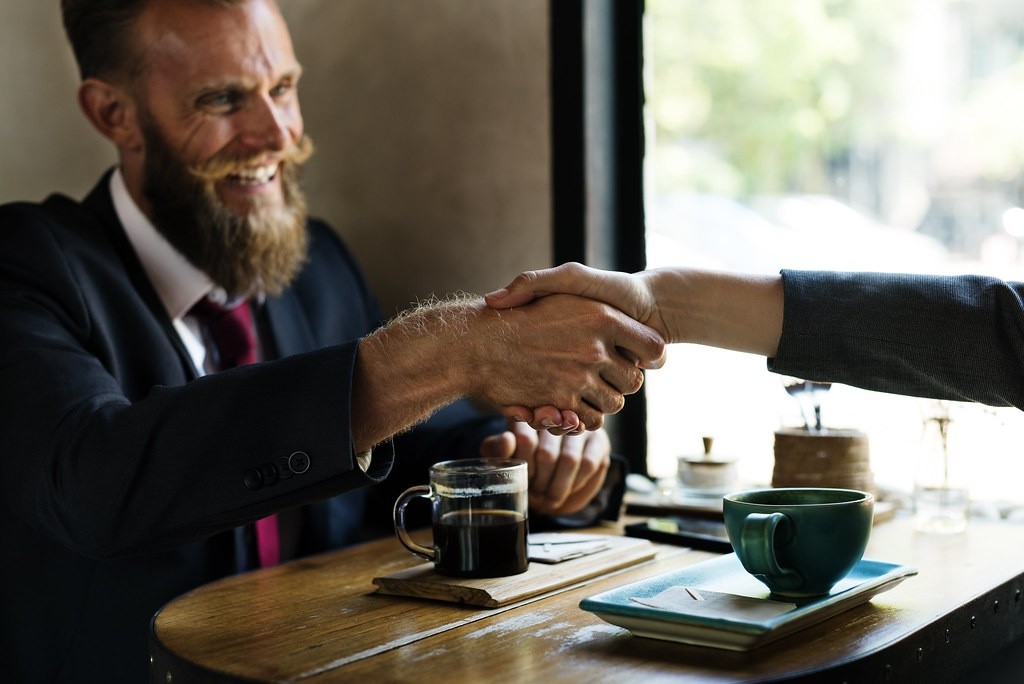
[393,458,528,578]
[723,487,874,597]
[912,484,968,535]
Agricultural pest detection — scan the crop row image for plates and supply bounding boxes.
[579,552,919,651]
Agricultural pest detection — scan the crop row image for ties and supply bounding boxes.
[191,298,280,569]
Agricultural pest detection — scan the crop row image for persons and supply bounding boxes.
[486,259,1024,436]
[0,0,668,684]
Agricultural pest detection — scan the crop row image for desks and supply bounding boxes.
[153,507,1024,682]
